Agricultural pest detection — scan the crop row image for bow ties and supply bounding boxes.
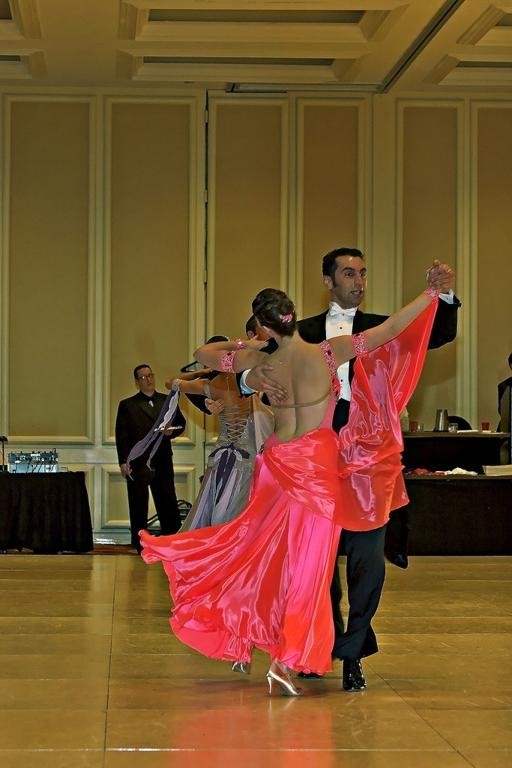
[329,301,359,317]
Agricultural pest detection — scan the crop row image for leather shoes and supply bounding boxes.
[342,661,366,692]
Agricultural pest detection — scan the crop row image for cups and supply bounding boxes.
[448,422,458,433]
[482,422,489,430]
[409,421,423,432]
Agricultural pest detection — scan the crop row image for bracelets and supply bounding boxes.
[232,337,246,350]
[423,286,437,298]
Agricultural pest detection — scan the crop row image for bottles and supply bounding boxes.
[434,409,450,432]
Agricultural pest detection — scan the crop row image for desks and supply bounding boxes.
[383,429,512,569]
[0,470,94,552]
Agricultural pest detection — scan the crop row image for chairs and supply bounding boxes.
[432,416,471,431]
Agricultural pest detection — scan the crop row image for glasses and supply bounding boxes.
[138,373,154,380]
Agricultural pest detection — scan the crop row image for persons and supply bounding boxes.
[191,264,452,696]
[165,334,274,536]
[189,316,271,413]
[235,248,460,691]
[115,363,186,556]
[496,353,511,433]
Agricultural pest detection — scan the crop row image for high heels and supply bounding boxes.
[267,670,304,697]
